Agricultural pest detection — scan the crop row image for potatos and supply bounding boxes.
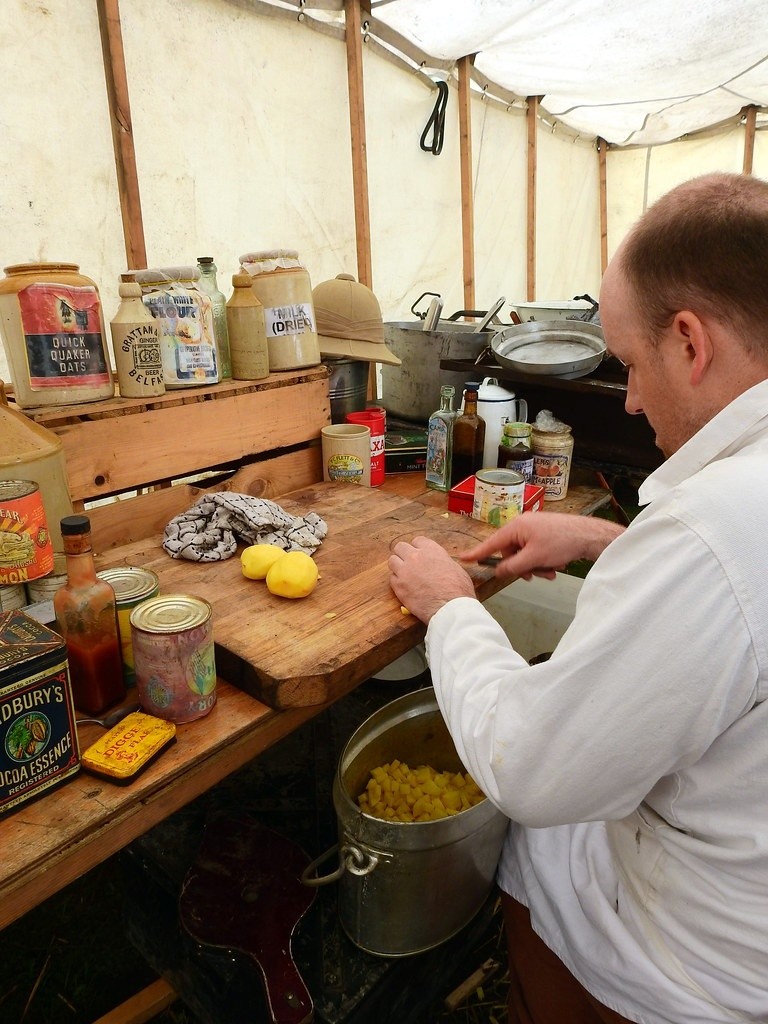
[401,607,409,614]
[358,758,486,823]
[241,544,318,598]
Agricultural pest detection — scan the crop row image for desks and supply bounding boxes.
[0,481,622,944]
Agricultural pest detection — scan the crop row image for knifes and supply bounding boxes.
[448,557,554,572]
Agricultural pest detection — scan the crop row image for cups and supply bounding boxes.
[321,424,370,489]
[345,412,386,488]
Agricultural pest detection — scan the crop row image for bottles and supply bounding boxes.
[0,262,116,409]
[118,267,223,391]
[497,421,534,484]
[425,386,460,492]
[193,257,233,379]
[109,274,166,399]
[53,515,128,718]
[226,274,270,381]
[450,382,486,489]
[239,249,321,373]
[531,421,574,501]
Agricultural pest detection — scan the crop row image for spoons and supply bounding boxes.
[76,704,142,729]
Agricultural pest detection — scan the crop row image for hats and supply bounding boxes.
[311,272,403,367]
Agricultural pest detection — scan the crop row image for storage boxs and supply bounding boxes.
[446,473,547,519]
[0,606,83,823]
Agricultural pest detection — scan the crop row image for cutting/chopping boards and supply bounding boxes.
[91,478,523,710]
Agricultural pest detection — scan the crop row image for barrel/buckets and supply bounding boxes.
[320,357,371,425]
[299,681,508,960]
[1,479,216,725]
[380,321,497,425]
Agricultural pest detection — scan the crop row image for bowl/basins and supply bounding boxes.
[509,300,593,323]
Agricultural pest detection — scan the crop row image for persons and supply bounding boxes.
[388,172,768,1024]
